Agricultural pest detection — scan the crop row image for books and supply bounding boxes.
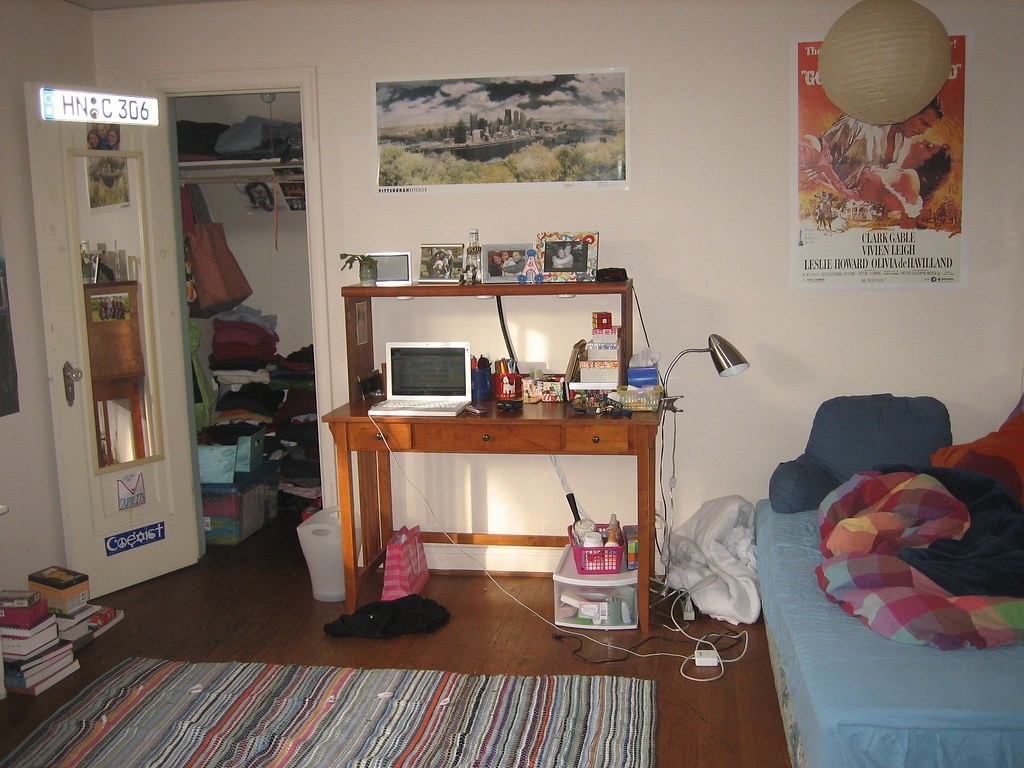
[0,565,125,697]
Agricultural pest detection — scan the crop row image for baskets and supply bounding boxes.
[567,523,627,575]
[617,385,663,412]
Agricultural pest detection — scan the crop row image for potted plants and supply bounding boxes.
[339,253,378,287]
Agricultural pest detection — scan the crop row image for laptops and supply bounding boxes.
[369,342,472,416]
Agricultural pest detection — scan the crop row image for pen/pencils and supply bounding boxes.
[497,357,516,373]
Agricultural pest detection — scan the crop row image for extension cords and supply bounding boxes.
[679,598,696,621]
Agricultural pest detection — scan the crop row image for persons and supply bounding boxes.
[551,244,574,268]
[99,295,126,320]
[426,249,454,279]
[489,251,526,276]
[88,124,120,151]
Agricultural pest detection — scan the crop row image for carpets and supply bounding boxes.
[0,659,656,768]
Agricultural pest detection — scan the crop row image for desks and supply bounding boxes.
[322,397,663,633]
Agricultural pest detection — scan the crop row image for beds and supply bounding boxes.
[756,498,1023,768]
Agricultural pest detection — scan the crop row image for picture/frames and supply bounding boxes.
[418,243,464,284]
[481,242,534,284]
[89,256,115,284]
[535,232,598,282]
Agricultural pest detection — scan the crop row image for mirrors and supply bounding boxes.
[67,150,165,475]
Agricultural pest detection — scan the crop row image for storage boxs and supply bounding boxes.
[552,545,640,630]
[586,342,621,360]
[234,428,265,475]
[623,525,638,570]
[568,379,619,404]
[202,458,284,545]
[28,565,91,611]
[578,360,620,383]
[538,376,565,403]
[592,325,622,342]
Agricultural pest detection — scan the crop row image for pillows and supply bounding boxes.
[768,393,951,515]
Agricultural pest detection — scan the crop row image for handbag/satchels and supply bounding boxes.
[382,524,430,601]
[180,184,253,319]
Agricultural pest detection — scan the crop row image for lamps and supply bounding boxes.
[661,333,750,409]
